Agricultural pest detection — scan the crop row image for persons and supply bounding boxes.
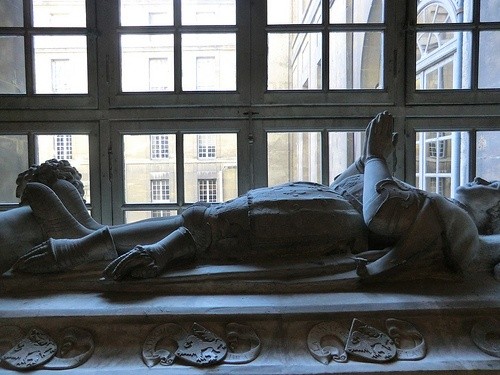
[11,108,499,283]
